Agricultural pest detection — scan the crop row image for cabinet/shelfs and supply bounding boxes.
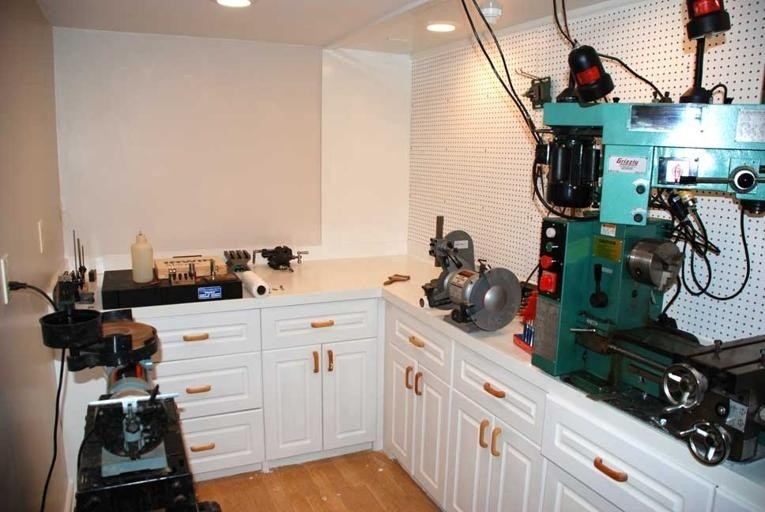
[262,299,373,470]
[371,298,763,507]
[53,310,261,492]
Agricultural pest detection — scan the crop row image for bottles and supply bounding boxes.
[131,234,154,283]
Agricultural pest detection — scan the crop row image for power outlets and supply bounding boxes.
[0,254,13,305]
